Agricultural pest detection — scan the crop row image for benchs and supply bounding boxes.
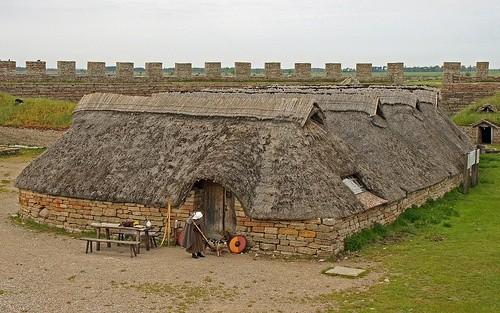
[80,237,141,258]
[113,231,161,249]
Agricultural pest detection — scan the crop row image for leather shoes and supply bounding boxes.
[192,254,198,259]
[197,253,205,257]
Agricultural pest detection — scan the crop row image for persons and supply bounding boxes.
[180,211,206,259]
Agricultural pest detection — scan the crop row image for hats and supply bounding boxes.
[191,212,203,219]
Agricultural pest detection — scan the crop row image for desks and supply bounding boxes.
[91,222,155,254]
